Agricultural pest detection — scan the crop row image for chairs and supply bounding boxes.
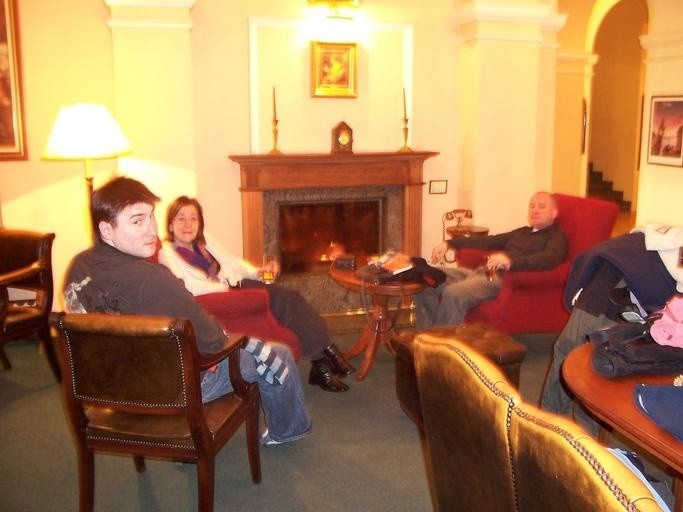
[147,238,302,369]
[0,222,63,389]
[39,310,263,512]
[403,332,675,512]
[451,188,620,335]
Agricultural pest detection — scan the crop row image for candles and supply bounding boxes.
[402,88,406,118]
[272,87,278,120]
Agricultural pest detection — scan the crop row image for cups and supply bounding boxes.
[261,254,275,285]
[481,254,496,283]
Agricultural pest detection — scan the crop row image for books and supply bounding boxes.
[381,256,413,274]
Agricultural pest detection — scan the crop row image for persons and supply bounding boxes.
[158,196,356,392]
[413,192,568,329]
[63,178,312,446]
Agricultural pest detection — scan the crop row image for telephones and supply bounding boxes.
[446,209,472,231]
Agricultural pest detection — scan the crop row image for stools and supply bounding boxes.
[385,319,527,430]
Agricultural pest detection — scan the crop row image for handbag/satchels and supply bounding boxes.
[357,262,413,285]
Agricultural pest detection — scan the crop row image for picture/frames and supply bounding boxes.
[0,0,31,163]
[308,37,358,101]
[645,93,682,167]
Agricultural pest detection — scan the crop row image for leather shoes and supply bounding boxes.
[309,358,349,392]
[324,341,355,377]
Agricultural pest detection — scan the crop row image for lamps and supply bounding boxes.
[39,100,130,250]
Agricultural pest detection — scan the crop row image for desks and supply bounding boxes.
[444,224,489,238]
[562,323,682,512]
[330,262,429,381]
